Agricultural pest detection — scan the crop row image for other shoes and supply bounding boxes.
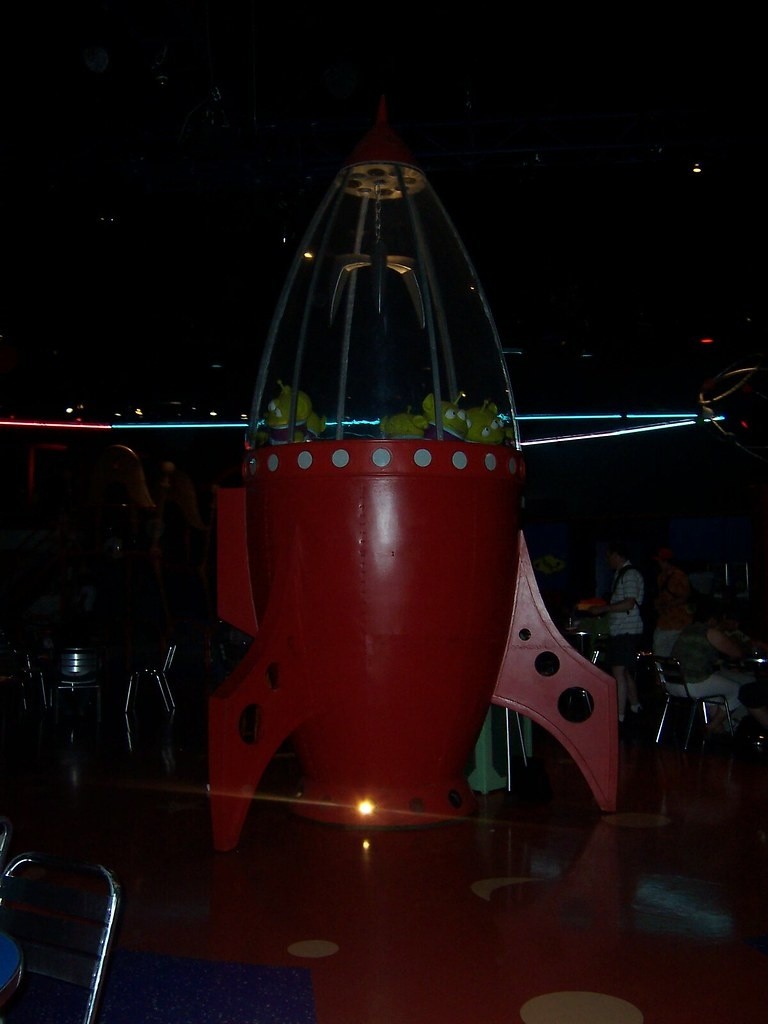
[625,709,649,723]
[704,722,725,734]
[618,720,628,732]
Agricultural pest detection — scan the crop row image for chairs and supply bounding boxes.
[0,852,125,1024]
[637,655,734,751]
[122,639,179,713]
[40,644,106,725]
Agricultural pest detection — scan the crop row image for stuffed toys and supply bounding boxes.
[379,391,515,445]
[255,380,327,446]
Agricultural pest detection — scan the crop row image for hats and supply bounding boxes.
[654,547,673,560]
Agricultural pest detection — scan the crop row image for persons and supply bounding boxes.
[651,547,706,690]
[661,609,768,843]
[589,540,646,725]
